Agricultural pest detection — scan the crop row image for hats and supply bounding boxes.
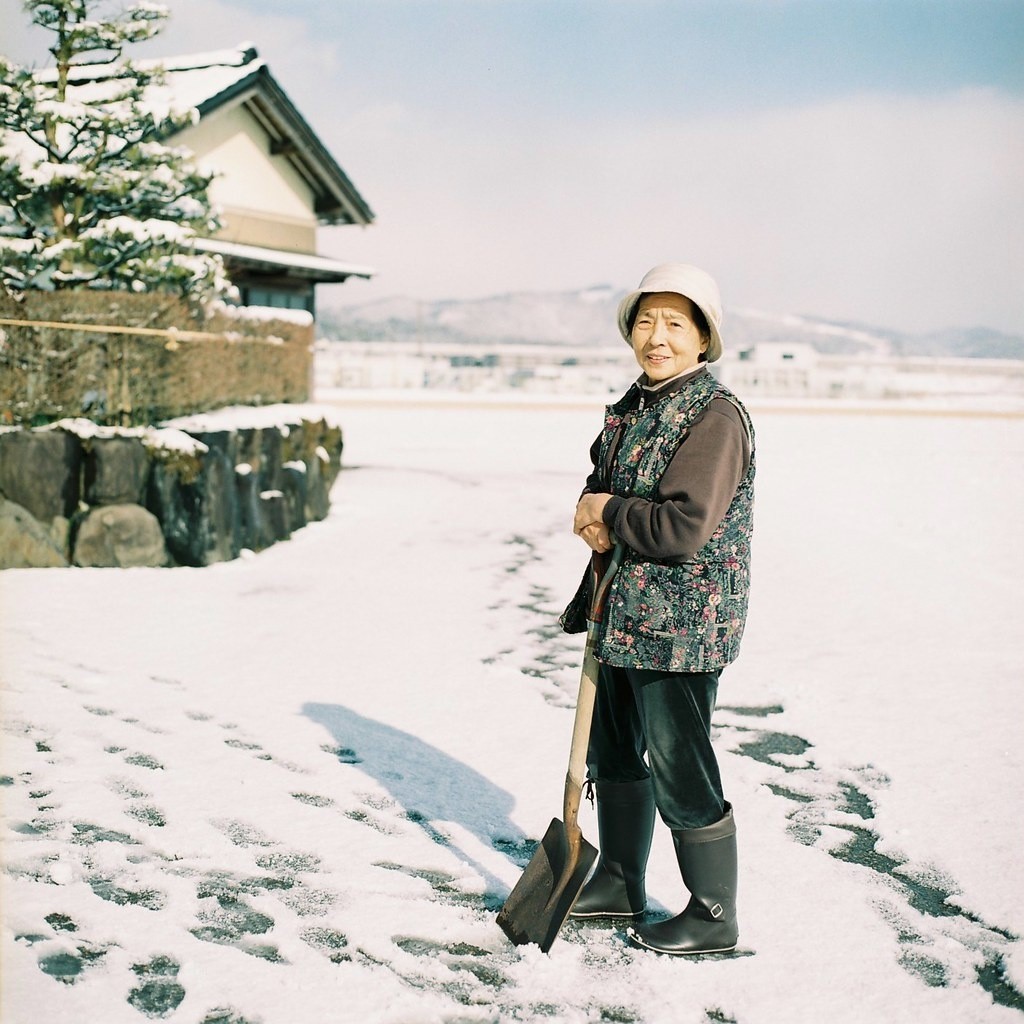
[617,262,722,363]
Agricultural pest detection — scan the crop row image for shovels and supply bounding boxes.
[495,543,624,954]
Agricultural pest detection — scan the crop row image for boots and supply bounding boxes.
[627,800,738,955]
[568,779,656,921]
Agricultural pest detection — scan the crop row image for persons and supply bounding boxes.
[558,263,758,957]
